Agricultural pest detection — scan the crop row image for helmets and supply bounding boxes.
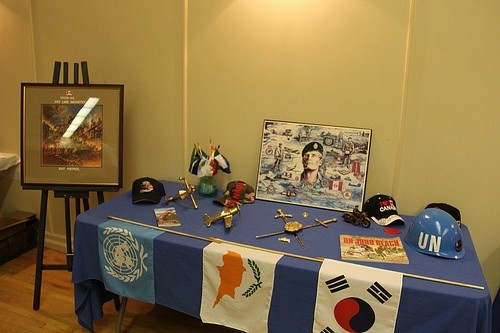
[406,209,466,263]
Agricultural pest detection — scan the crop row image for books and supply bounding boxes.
[339,234,410,265]
[153,207,181,227]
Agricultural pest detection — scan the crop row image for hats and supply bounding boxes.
[212,178,255,207]
[130,176,166,206]
[424,203,462,232]
[362,193,405,227]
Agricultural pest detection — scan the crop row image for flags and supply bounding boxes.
[98,219,166,305]
[313,259,403,333]
[188,145,232,178]
[200,242,284,333]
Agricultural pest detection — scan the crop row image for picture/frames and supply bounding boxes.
[20,83,125,190]
[257,119,372,214]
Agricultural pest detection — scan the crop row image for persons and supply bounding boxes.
[353,205,362,224]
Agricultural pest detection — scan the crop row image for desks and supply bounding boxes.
[72,180,492,333]
